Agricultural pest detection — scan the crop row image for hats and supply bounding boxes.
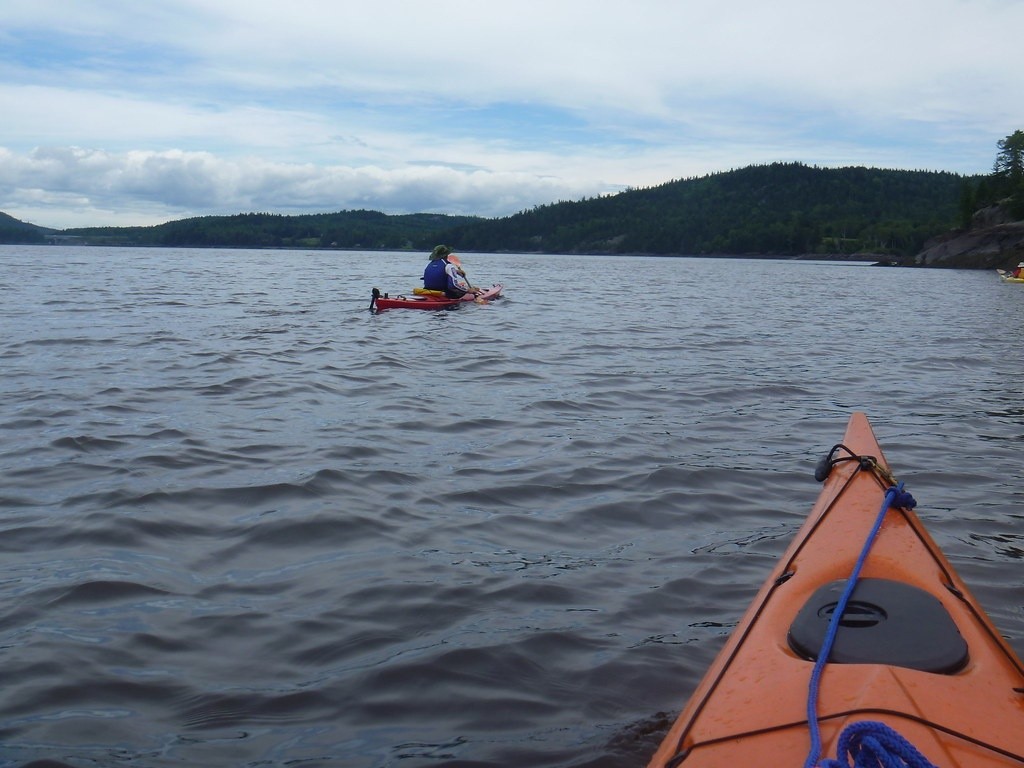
[1018,262,1024,268]
[429,245,454,260]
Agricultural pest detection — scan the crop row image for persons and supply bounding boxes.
[1012,262,1024,278]
[424,245,475,301]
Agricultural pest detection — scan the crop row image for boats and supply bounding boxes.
[997,268,1024,282]
[368,283,505,314]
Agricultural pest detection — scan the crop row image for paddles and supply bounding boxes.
[446,254,488,306]
